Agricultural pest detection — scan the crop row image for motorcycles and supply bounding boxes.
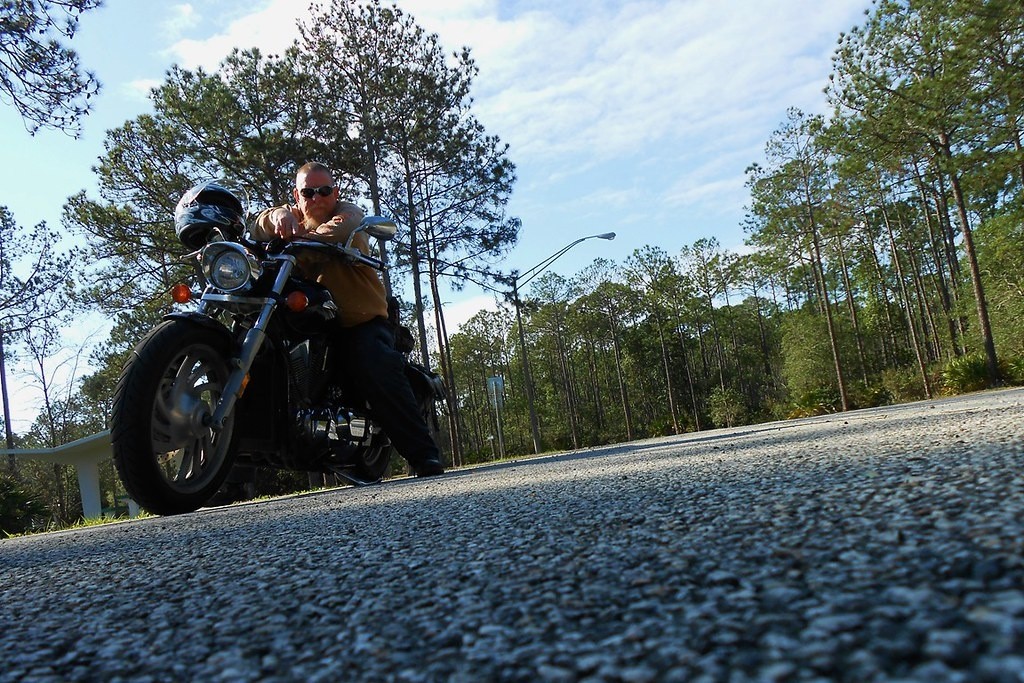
[109,216,449,517]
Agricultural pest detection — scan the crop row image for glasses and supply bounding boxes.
[296,184,337,198]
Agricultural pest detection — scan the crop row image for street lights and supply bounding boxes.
[512,232,616,455]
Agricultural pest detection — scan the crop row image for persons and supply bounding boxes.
[247,161,444,486]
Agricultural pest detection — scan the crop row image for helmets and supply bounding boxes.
[174,183,246,251]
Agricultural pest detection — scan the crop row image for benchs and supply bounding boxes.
[117,495,140,517]
[103,505,129,518]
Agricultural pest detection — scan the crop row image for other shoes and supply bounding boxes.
[416,458,444,477]
